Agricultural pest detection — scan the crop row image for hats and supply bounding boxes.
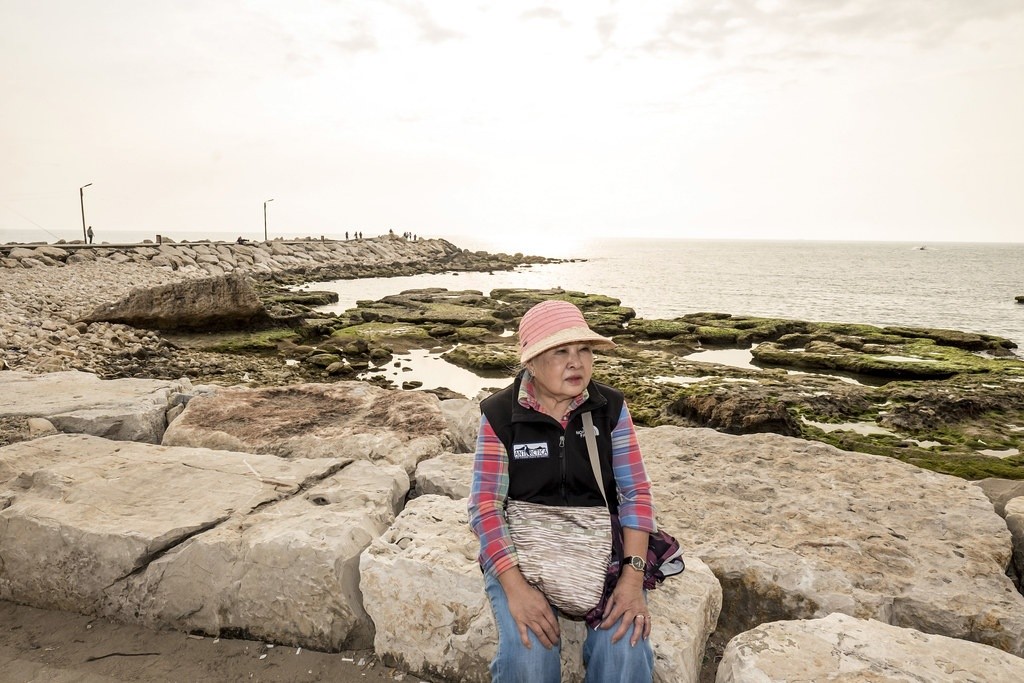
[519,299,618,364]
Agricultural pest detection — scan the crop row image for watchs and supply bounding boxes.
[623,555,647,571]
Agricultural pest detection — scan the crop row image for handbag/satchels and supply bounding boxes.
[506,498,612,617]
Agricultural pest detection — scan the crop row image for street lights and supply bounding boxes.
[264,199,275,240]
[80,183,92,243]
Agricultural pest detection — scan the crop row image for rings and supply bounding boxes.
[637,615,644,618]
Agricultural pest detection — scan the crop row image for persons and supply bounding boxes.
[404,231,411,240]
[87,226,95,244]
[345,231,362,240]
[467,300,654,683]
